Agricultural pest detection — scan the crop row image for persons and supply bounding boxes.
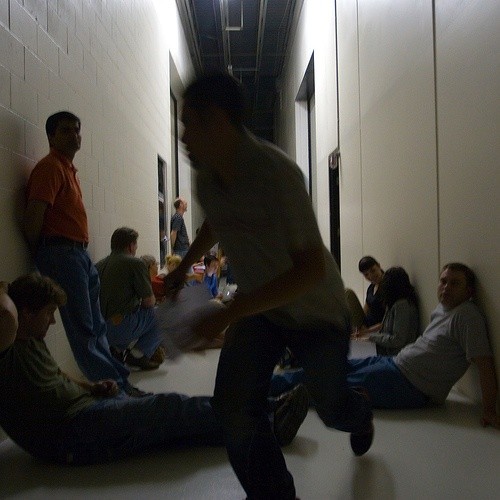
[1,270,310,500]
[166,66,375,500]
[268,252,499,428]
[95,196,237,371]
[20,111,154,399]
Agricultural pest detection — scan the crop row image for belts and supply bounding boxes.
[42,232,90,250]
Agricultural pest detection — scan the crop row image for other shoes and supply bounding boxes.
[125,383,154,397]
[347,387,374,456]
[269,383,310,448]
[139,353,159,369]
